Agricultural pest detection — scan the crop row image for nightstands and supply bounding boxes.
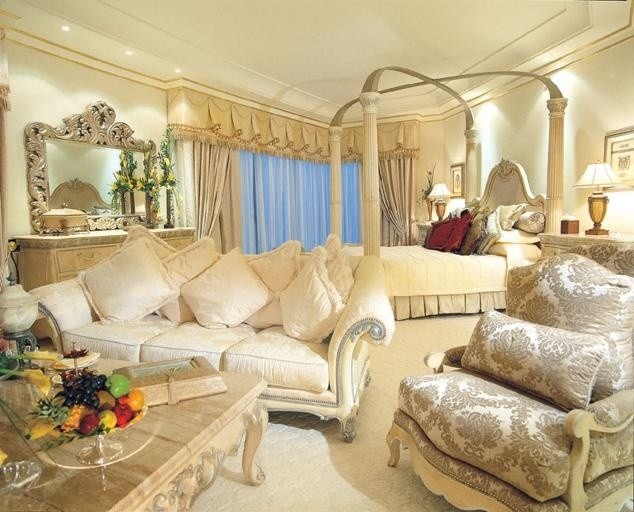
[414,223,428,244]
[536,231,634,261]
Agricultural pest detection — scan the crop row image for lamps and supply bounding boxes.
[426,184,453,220]
[572,160,625,235]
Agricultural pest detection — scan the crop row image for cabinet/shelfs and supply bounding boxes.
[13,225,195,292]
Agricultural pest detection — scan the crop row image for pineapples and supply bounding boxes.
[60,404,92,430]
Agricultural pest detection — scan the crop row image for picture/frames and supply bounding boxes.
[449,163,465,199]
[601,127,634,192]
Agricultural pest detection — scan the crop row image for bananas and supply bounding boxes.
[26,416,56,440]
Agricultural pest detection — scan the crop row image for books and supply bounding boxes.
[113,355,227,409]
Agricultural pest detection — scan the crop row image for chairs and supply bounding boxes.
[385,244,634,511]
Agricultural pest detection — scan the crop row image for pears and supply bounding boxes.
[98,409,117,431]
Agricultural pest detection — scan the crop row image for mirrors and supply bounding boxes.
[23,98,159,230]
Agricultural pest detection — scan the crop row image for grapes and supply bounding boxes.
[64,369,106,408]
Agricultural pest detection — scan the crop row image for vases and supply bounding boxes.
[110,196,121,216]
[164,191,176,228]
[426,199,434,220]
[143,192,158,227]
[122,190,135,215]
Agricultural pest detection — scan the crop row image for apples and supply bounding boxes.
[113,404,134,426]
[81,412,99,432]
[95,391,116,411]
[105,373,130,399]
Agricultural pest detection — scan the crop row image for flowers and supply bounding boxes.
[136,145,158,197]
[108,151,136,194]
[158,134,178,190]
[417,162,438,206]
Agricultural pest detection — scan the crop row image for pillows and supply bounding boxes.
[278,244,347,344]
[115,226,180,316]
[240,240,301,329]
[76,236,181,325]
[180,246,274,331]
[459,312,611,411]
[423,201,545,261]
[321,234,355,299]
[157,237,221,327]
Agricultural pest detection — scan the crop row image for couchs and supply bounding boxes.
[28,223,394,443]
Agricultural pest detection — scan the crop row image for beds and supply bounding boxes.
[50,177,110,210]
[342,154,547,321]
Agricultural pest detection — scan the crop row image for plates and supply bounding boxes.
[51,352,101,370]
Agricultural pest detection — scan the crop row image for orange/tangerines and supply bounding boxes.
[118,389,145,412]
